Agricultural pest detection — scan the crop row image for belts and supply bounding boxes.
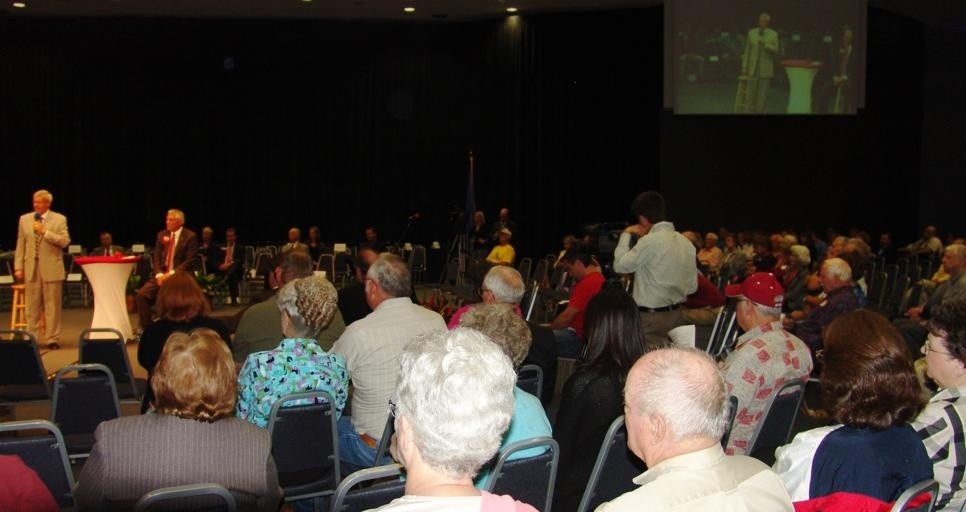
[638,301,681,312]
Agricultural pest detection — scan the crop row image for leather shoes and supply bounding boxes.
[47,342,60,350]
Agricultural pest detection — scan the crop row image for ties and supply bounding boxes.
[226,244,233,264]
[164,233,174,274]
[106,246,110,255]
[35,217,43,259]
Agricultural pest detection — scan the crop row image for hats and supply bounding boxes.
[724,270,786,308]
[500,227,513,237]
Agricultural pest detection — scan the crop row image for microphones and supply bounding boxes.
[34,212,40,233]
[409,213,420,220]
[758,28,763,45]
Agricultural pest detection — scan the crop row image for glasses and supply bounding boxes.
[921,339,953,355]
[815,348,825,364]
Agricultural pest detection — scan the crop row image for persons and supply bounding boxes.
[740,12,782,112]
[814,27,865,113]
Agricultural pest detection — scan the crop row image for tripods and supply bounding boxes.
[439,224,472,288]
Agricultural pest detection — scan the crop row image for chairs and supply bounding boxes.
[0,236,942,511]
